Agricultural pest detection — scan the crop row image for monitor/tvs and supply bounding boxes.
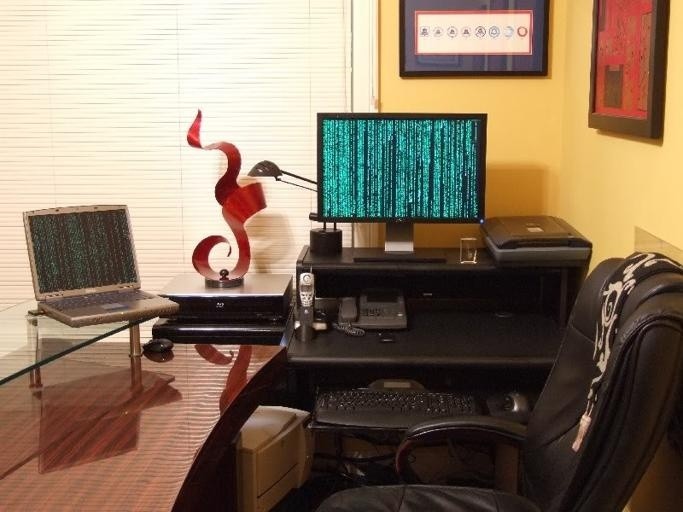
[316,112,486,262]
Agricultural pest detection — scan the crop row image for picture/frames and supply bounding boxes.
[588,1,670,138]
[398,0,549,77]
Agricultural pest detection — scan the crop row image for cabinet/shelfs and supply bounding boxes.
[152,274,293,346]
[232,403,311,510]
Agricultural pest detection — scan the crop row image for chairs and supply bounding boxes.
[319,250,683,512]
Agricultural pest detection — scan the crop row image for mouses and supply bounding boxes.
[502,388,528,412]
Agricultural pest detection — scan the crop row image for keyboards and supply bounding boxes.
[316,378,479,436]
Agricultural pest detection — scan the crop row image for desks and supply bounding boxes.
[286,244,590,411]
[0,298,286,511]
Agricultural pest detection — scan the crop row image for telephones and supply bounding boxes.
[297,265,314,342]
[338,292,408,337]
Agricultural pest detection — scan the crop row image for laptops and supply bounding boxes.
[22,204,181,328]
